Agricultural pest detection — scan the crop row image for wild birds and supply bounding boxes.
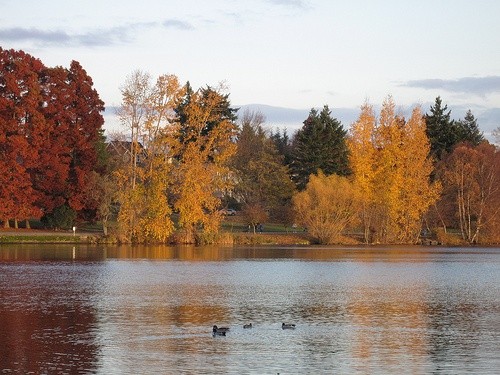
[282,323,296,329]
[243,323,253,329]
[213,325,230,333]
